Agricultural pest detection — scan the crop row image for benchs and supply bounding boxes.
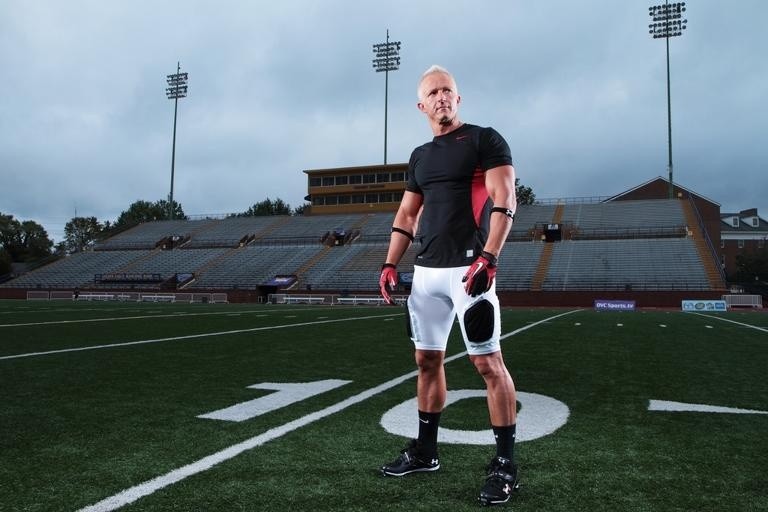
[0,197,723,299]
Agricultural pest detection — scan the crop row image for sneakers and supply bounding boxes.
[380,440,440,476]
[477,456,520,504]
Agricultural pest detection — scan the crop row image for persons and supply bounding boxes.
[73,287,80,303]
[379,65,518,506]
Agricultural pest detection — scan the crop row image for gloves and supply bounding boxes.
[461,250,498,297]
[379,264,399,307]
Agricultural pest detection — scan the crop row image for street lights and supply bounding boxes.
[372,28,401,165]
[648,0,687,199]
[165,60,188,220]
